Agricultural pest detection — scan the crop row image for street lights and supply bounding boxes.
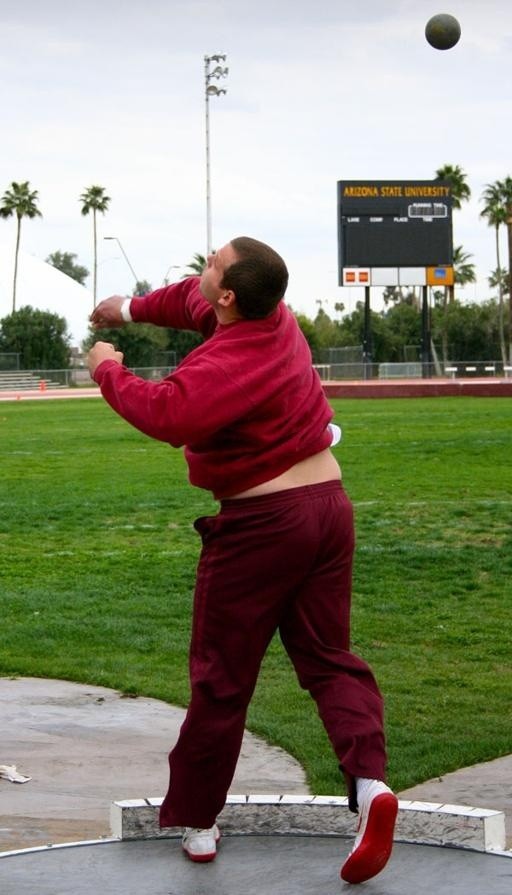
[159,264,180,288]
[103,236,143,298]
[204,51,228,262]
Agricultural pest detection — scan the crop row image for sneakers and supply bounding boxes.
[341,779,400,883]
[182,823,220,862]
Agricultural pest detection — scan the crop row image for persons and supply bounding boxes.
[82,233,404,887]
